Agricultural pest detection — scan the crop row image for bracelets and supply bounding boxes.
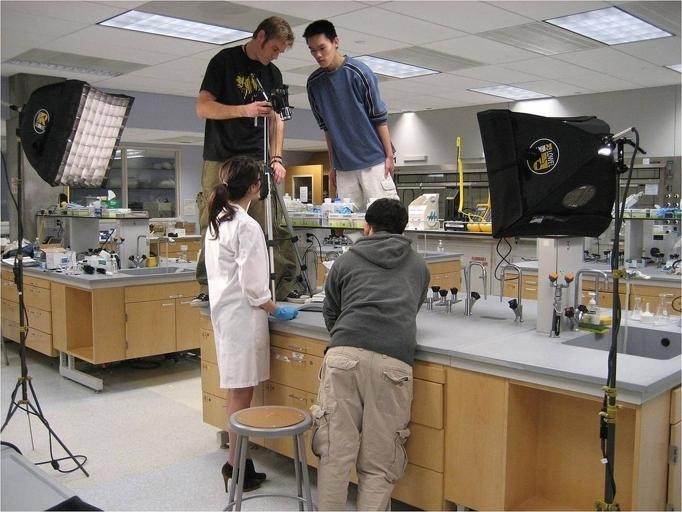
[269,155,283,164]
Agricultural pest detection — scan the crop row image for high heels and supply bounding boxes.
[221,459,267,492]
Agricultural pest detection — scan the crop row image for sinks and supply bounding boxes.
[562,325,682,360]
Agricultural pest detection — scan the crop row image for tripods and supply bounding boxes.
[245,114,314,316]
[0,140,89,477]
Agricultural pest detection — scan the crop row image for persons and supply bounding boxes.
[309,198,431,510]
[304,19,401,250]
[192,15,294,307]
[203,155,297,492]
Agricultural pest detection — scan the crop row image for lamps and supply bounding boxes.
[17,76,136,188]
[476,110,617,239]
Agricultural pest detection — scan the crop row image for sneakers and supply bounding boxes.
[284,290,306,303]
[190,292,209,307]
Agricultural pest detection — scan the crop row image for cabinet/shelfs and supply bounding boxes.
[2,266,124,367]
[200,314,444,510]
[150,240,201,262]
[502,273,538,299]
[445,350,681,511]
[581,280,681,316]
[425,258,461,297]
[125,280,200,361]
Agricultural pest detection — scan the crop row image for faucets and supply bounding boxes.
[465,263,488,316]
[573,267,609,311]
[500,263,523,321]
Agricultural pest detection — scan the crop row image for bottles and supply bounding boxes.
[631,297,654,324]
[96,260,107,274]
[321,197,353,227]
[178,244,188,265]
[149,237,169,267]
[32,237,42,263]
[619,190,644,210]
[641,247,646,268]
[283,192,291,205]
[656,293,670,323]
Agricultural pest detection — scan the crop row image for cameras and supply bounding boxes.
[268,85,292,121]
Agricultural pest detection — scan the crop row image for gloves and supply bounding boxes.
[274,306,299,321]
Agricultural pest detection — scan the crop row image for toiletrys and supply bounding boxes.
[437,240,444,253]
